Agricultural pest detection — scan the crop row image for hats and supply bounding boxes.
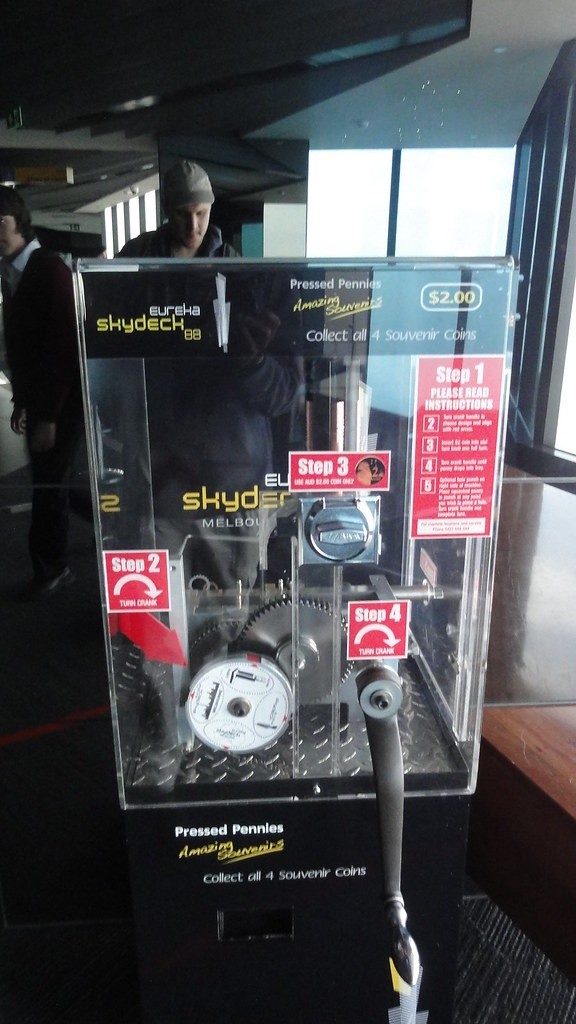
[162,162,215,207]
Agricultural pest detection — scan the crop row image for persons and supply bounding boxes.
[117,160,241,257]
[87,266,303,754]
[60,232,107,269]
[0,185,93,601]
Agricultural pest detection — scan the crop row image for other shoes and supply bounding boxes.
[27,564,69,594]
